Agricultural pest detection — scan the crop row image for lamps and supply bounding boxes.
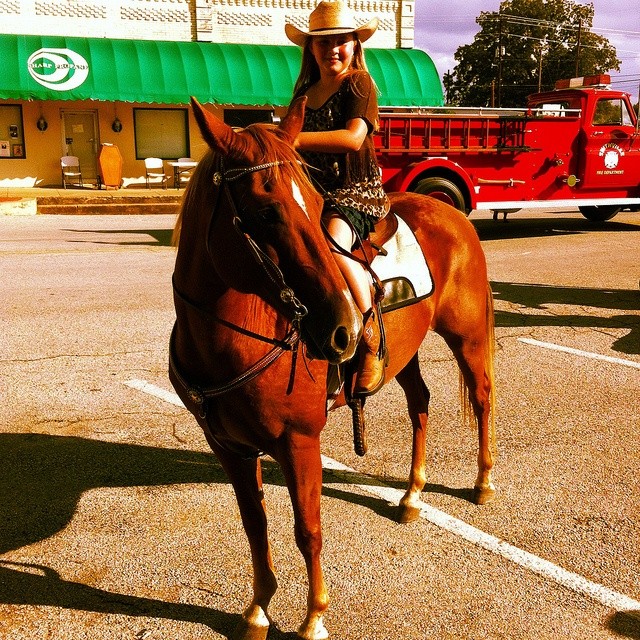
[37,116,48,132]
[111,118,122,132]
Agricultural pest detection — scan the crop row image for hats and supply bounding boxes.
[285,2,380,46]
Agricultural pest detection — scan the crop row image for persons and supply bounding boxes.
[283,3,390,397]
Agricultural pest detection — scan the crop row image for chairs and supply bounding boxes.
[60,156,83,189]
[144,158,168,190]
[178,158,193,175]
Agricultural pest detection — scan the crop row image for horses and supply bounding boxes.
[168,95,494,640]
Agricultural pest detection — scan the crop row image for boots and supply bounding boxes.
[353,307,389,400]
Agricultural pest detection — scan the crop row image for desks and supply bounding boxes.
[167,162,198,189]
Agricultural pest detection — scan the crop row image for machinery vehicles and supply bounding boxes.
[373,74,640,225]
